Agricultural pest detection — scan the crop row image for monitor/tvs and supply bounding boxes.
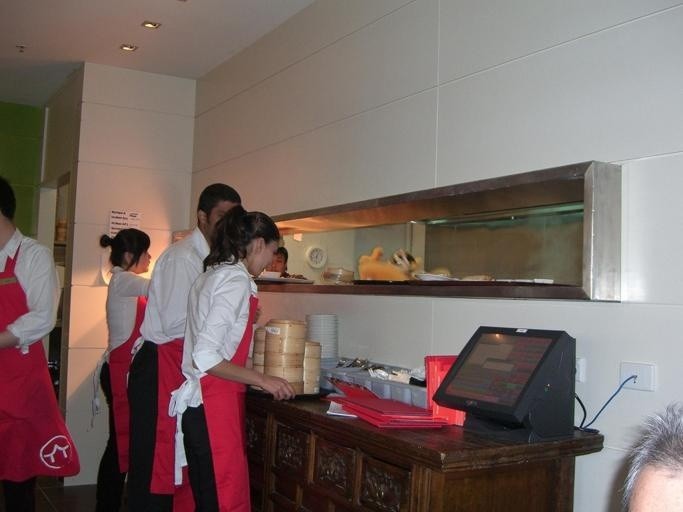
[431,325,574,424]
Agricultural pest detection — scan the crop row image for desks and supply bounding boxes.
[243,386,605,512]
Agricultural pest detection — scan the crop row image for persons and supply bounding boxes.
[266,247,290,278]
[0,176,79,512]
[169,205,296,512]
[96,229,152,512]
[610,401,683,512]
[126,184,241,512]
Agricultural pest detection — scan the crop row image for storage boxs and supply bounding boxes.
[316,356,428,410]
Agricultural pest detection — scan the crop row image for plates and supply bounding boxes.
[304,314,339,368]
[414,273,451,281]
